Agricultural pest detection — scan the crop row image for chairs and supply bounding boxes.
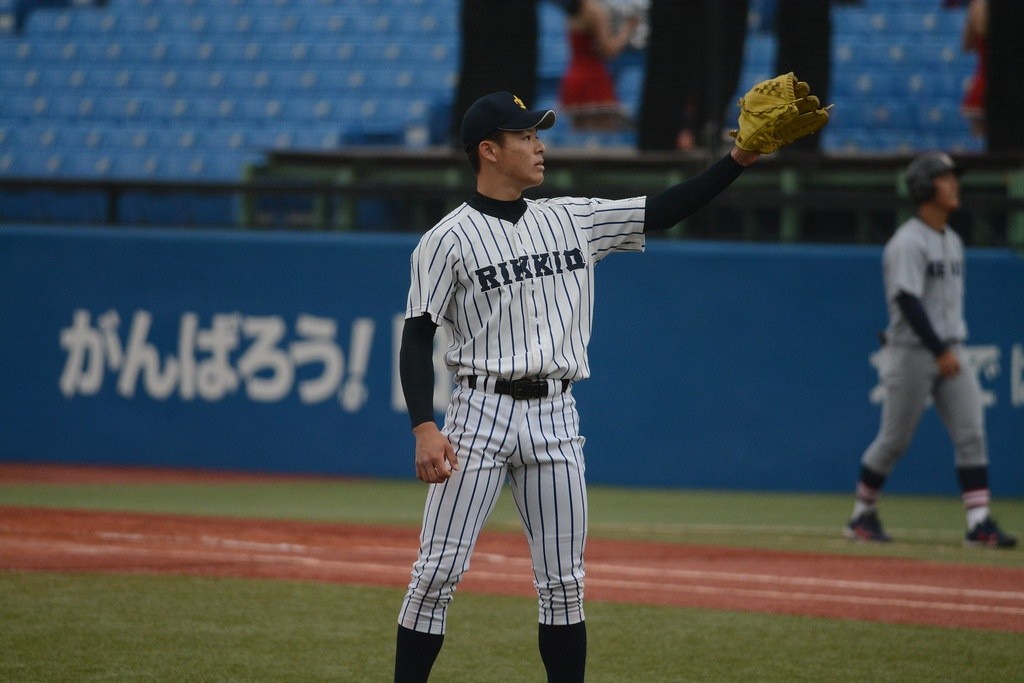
[0,1,983,230]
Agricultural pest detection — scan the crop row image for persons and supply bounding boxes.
[392,72,829,682]
[961,0,1024,169]
[559,0,640,131]
[637,0,749,152]
[843,150,1019,549]
[770,0,860,156]
[451,0,539,138]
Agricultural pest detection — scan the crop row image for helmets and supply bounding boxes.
[905,150,959,203]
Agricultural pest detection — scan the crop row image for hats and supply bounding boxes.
[460,91,556,153]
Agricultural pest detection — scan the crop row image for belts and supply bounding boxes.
[468,374,571,401]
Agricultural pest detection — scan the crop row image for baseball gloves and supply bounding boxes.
[733,69,829,153]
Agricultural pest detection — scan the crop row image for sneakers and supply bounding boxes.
[844,509,888,542]
[963,518,1015,549]
